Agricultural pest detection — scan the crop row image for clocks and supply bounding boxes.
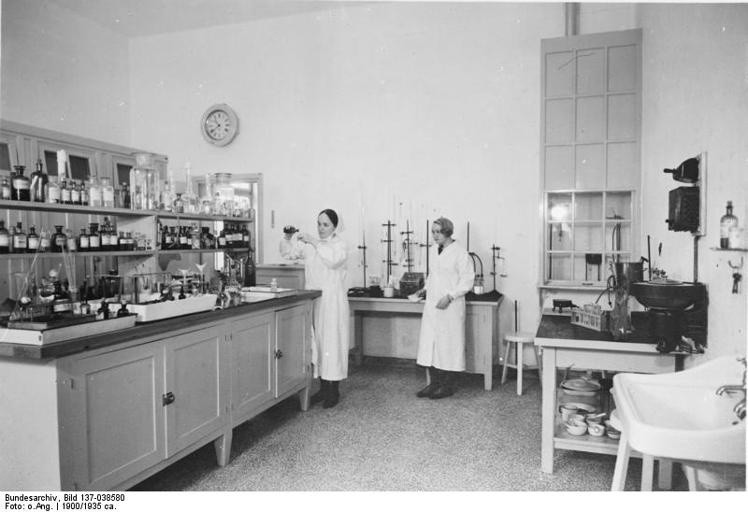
[201,104,239,146]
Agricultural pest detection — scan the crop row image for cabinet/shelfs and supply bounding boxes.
[0,198,255,259]
[229,300,313,429]
[54,318,227,491]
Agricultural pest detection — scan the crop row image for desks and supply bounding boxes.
[348,295,503,391]
[535,314,689,490]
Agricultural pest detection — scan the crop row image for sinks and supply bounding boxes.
[611,355,746,491]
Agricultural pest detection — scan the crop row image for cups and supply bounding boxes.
[366,284,394,298]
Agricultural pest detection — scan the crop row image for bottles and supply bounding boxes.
[473,274,483,294]
[611,225,621,251]
[718,199,748,250]
[584,302,602,329]
[3,145,256,320]
[282,226,299,234]
[269,277,278,293]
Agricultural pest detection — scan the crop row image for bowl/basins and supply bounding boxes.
[559,376,623,440]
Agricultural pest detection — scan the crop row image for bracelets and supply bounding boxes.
[447,294,452,303]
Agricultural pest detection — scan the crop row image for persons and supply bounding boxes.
[279,208,351,409]
[414,217,476,399]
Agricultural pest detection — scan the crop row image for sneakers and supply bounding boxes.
[311,391,340,408]
[417,382,458,399]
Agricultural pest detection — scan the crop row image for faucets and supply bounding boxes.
[715,369,746,396]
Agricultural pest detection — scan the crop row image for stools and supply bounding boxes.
[611,410,697,491]
[501,333,542,396]
